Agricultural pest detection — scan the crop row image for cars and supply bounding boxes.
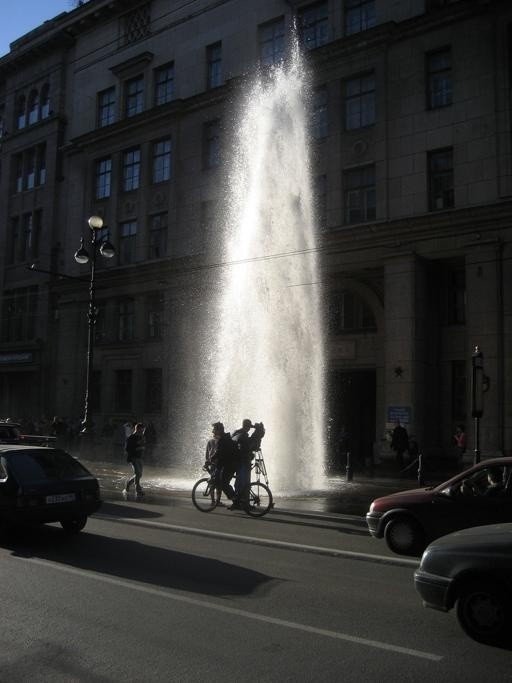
[1,444,102,534]
[413,522,512,648]
[366,456,512,558]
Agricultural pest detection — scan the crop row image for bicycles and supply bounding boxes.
[191,461,274,517]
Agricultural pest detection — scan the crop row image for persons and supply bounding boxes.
[390,419,409,470]
[460,463,503,498]
[202,421,247,510]
[205,435,225,507]
[123,421,148,496]
[453,424,467,459]
[228,418,263,510]
[0,411,158,463]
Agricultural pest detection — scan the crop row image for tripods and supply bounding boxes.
[250,451,275,511]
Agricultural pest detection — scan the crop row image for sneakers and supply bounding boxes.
[122,488,129,495]
[134,484,143,492]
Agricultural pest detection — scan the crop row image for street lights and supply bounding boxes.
[72,214,116,437]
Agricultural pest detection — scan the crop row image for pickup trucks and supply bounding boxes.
[0,420,58,449]
